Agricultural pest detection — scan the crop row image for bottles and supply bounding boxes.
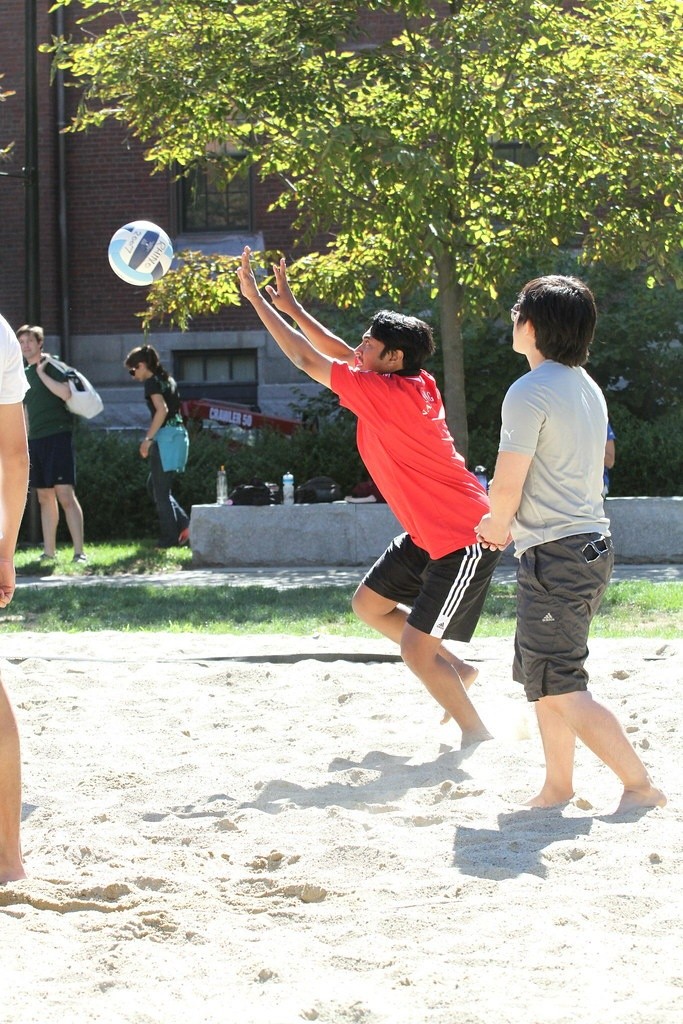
[216,464,227,503]
[283,471,294,505]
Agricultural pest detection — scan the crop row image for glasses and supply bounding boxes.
[510,303,524,321]
[128,364,138,377]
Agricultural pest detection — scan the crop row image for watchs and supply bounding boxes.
[145,437,153,441]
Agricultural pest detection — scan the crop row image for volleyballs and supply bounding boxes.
[107,219,174,287]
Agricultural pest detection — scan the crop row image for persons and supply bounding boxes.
[124,345,190,549]
[15,325,87,564]
[472,273,666,817]
[0,310,32,886]
[235,245,514,749]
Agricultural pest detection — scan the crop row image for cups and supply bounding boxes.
[475,465,487,491]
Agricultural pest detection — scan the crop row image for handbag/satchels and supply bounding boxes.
[46,355,104,420]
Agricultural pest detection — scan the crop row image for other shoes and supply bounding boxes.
[37,553,56,563]
[71,553,87,564]
[155,546,160,552]
[178,527,189,545]
